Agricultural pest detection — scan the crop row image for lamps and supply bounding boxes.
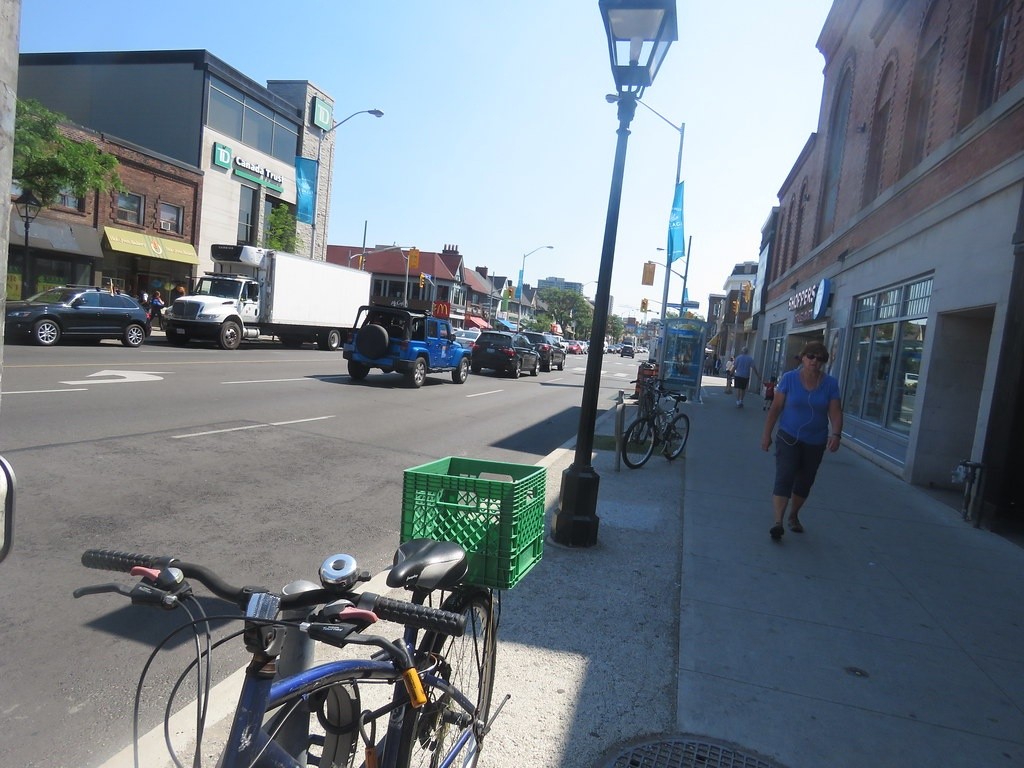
[856,122,866,133]
[836,249,849,263]
[804,193,810,201]
[790,281,798,290]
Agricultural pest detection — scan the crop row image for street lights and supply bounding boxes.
[13,186,43,301]
[311,109,385,263]
[656,235,693,317]
[548,0,679,551]
[517,246,554,335]
[604,94,686,404]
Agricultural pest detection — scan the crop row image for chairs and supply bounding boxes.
[390,325,402,338]
[417,325,424,340]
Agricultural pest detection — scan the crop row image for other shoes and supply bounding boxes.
[724,389,732,394]
[736,401,743,408]
[767,407,770,410]
[763,407,766,410]
[786,518,803,532]
[770,524,784,540]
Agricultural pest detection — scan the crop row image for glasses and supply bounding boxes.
[804,352,826,362]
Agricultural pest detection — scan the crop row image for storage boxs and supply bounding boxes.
[398,455,548,591]
[397,292,401,297]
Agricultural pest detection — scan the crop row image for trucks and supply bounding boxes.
[165,243,374,350]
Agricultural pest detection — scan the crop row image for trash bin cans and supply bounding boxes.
[635,361,655,398]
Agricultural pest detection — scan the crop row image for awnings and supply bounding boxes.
[466,317,493,330]
[102,227,201,264]
[9,210,105,262]
[497,319,517,331]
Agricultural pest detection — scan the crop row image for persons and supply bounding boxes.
[762,377,778,411]
[730,347,760,408]
[144,291,166,330]
[703,354,722,376]
[138,289,149,305]
[762,343,843,539]
[726,357,735,385]
[174,285,186,298]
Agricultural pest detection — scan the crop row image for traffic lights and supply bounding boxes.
[742,281,751,304]
[419,274,426,288]
[732,299,739,315]
[640,299,648,312]
[507,286,516,300]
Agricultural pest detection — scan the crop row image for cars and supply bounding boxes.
[550,335,649,356]
[4,283,154,347]
[439,327,482,350]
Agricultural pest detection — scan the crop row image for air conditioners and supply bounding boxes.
[160,220,170,231]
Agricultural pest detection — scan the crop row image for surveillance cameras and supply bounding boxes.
[606,94,618,103]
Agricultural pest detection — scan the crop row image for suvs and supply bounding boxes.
[343,304,472,392]
[621,345,635,358]
[515,329,568,373]
[471,329,542,379]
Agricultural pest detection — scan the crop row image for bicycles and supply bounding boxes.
[622,377,692,470]
[73,537,512,768]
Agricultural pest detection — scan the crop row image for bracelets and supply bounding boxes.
[832,432,842,439]
